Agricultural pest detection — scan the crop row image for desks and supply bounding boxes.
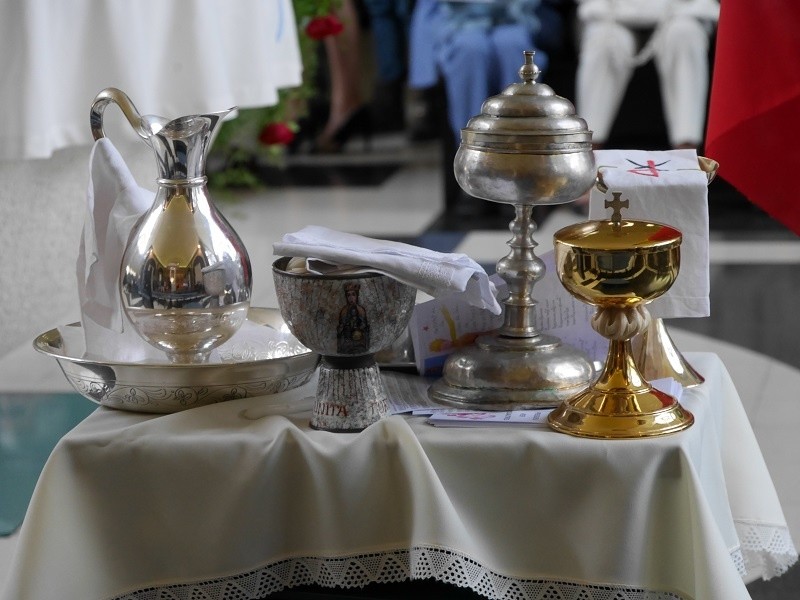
[62,351,717,600]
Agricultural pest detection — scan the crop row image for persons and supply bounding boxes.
[310,0,372,155]
[408,0,720,149]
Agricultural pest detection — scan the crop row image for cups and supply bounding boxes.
[271,257,416,432]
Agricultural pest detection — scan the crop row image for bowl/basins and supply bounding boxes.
[32,305,322,413]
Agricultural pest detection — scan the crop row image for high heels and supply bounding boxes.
[301,101,374,156]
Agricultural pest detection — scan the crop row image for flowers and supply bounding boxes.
[208,0,352,206]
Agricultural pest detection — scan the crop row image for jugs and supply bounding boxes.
[90,90,253,364]
